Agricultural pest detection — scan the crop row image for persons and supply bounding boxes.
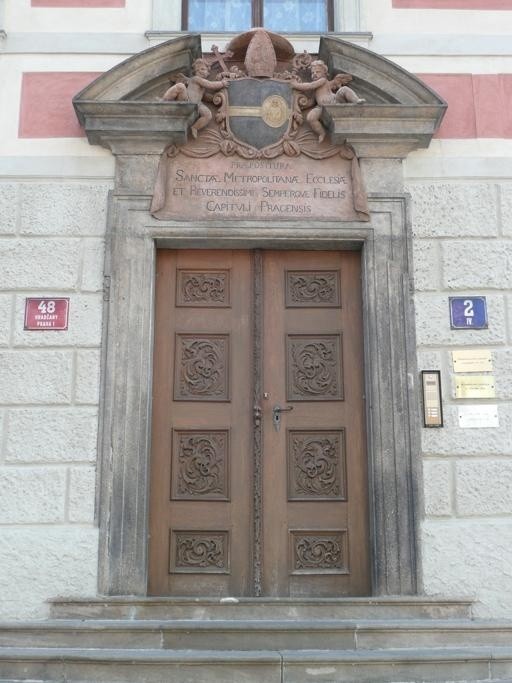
[156,58,229,140]
[292,59,366,144]
